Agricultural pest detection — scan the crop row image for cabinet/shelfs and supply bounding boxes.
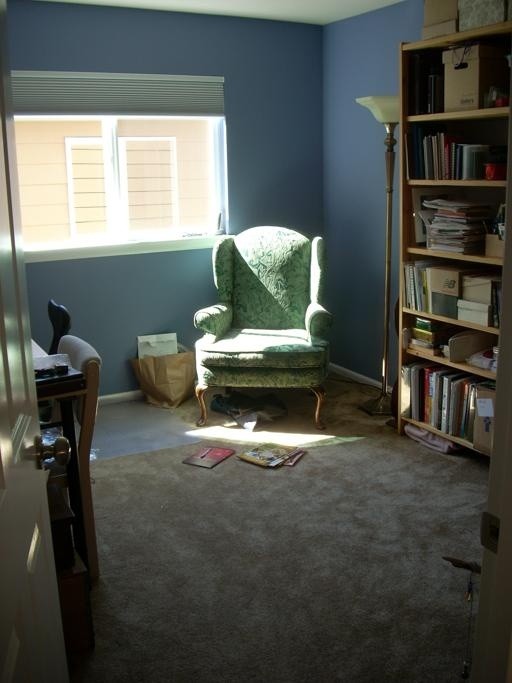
[399,19,512,459]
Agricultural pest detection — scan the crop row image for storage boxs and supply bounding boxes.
[485,233,505,258]
[429,265,501,327]
[472,386,497,456]
[441,45,508,113]
[422,0,512,40]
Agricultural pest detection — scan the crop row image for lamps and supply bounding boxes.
[354,93,400,419]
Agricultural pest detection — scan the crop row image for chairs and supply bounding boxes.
[57,335,102,590]
[193,226,333,427]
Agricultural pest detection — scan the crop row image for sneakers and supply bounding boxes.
[210,390,287,419]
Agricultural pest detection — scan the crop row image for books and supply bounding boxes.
[420,196,497,255]
[182,446,236,469]
[400,360,477,445]
[403,258,440,315]
[237,442,305,468]
[403,126,491,182]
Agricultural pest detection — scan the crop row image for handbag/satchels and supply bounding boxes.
[128,344,196,409]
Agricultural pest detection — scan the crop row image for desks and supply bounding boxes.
[30,339,95,665]
